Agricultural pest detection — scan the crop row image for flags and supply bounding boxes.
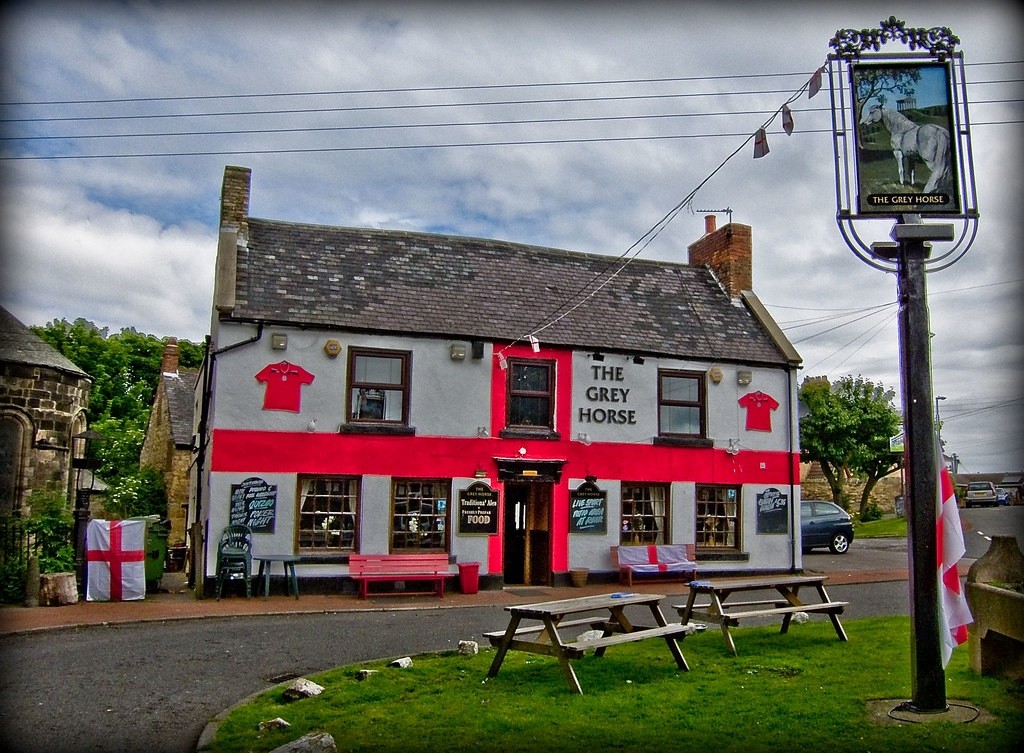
[86,519,147,601]
[618,545,697,574]
[935,430,973,671]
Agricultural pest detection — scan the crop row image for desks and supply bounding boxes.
[676,577,848,657]
[488,592,690,694]
[253,554,302,601]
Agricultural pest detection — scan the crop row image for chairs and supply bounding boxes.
[214,525,252,601]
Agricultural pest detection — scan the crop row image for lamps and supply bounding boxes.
[727,442,740,455]
[626,354,645,365]
[307,417,317,433]
[587,351,605,362]
[578,432,592,444]
[477,425,489,438]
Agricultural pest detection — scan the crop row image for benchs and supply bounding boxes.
[667,599,851,620]
[348,554,454,600]
[610,543,697,586]
[482,616,690,653]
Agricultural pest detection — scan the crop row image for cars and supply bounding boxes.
[995,488,1009,506]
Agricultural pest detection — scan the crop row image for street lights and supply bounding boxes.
[935,395,946,442]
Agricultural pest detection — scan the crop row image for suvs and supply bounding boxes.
[965,481,999,508]
[800,500,855,555]
